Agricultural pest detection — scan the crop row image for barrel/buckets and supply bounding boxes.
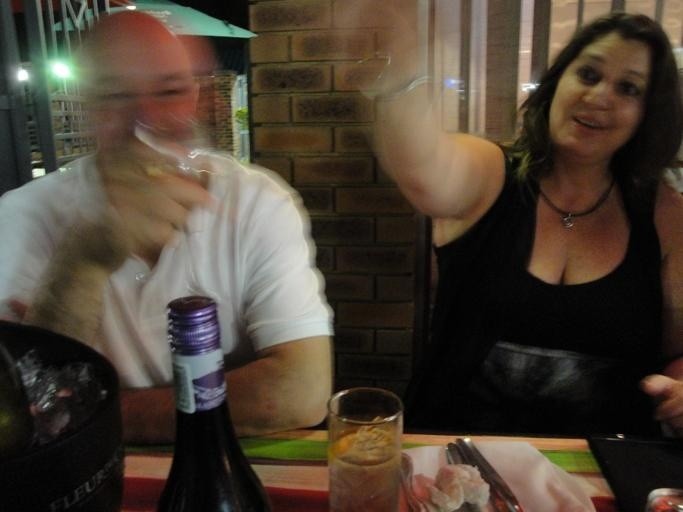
[1,323,125,512]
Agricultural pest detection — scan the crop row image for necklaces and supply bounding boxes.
[534,166,617,228]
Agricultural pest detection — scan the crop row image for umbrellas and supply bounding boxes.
[54,1,259,40]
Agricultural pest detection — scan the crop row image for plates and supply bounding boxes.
[402,439,596,512]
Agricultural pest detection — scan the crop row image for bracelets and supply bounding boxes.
[374,74,430,104]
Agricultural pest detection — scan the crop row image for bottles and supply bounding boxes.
[156,296,271,511]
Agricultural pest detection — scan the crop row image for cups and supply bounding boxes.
[326,387,403,509]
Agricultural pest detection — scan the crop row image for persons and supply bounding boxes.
[0,12,335,444]
[358,0,683,436]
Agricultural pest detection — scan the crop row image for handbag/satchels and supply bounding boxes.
[585,417,681,503]
